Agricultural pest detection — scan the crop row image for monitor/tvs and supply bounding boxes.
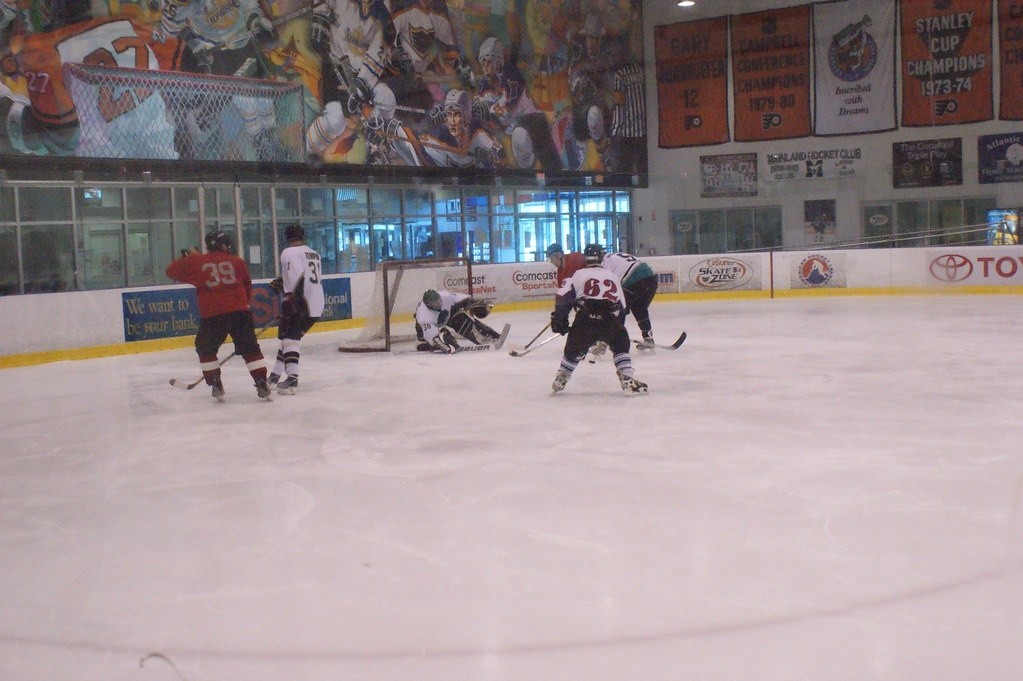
[75,187,102,207]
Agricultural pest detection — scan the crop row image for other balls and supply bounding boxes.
[589,360,595,364]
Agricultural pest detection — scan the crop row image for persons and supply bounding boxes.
[550,245,648,397]
[415,288,511,354]
[547,243,658,363]
[812,214,826,242]
[166,231,273,401]
[268,225,324,396]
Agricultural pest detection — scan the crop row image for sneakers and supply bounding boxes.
[589,341,607,358]
[277,375,297,395]
[211,376,225,400]
[636,338,654,353]
[618,368,649,397]
[552,372,569,396]
[256,377,272,401]
[267,373,279,388]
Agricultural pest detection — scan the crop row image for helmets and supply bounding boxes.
[423,290,443,308]
[584,244,600,263]
[205,230,230,252]
[285,224,305,241]
[546,244,562,258]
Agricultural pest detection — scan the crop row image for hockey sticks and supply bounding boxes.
[510,321,552,349]
[168,313,279,389]
[629,332,686,351]
[393,323,511,356]
[508,332,561,358]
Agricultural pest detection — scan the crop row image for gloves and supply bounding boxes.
[269,277,284,294]
[551,311,571,336]
[281,292,296,315]
